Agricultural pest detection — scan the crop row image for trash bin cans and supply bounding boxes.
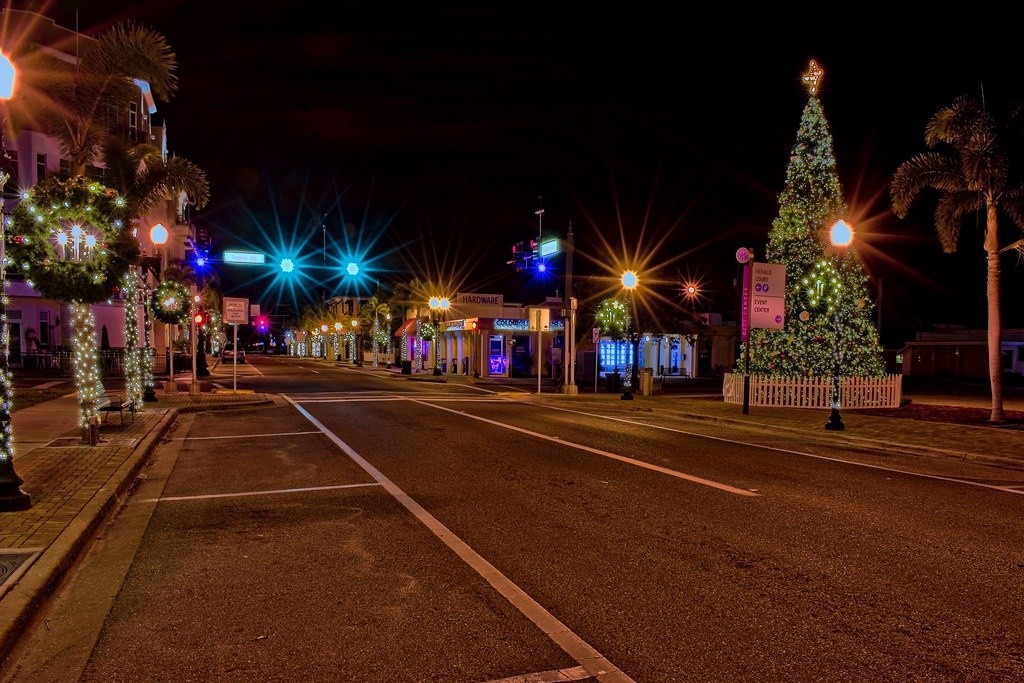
[337,354,341,361]
[644,368,653,395]
[401,360,412,374]
[166,351,181,373]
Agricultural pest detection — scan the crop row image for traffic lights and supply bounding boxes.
[345,262,360,276]
[195,314,203,324]
[279,258,296,274]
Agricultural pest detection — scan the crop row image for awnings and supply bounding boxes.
[395,316,429,337]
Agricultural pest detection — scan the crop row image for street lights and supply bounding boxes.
[130,224,168,402]
[320,323,358,364]
[824,220,853,429]
[621,272,637,399]
[429,297,449,376]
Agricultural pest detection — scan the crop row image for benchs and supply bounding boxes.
[100,392,135,429]
